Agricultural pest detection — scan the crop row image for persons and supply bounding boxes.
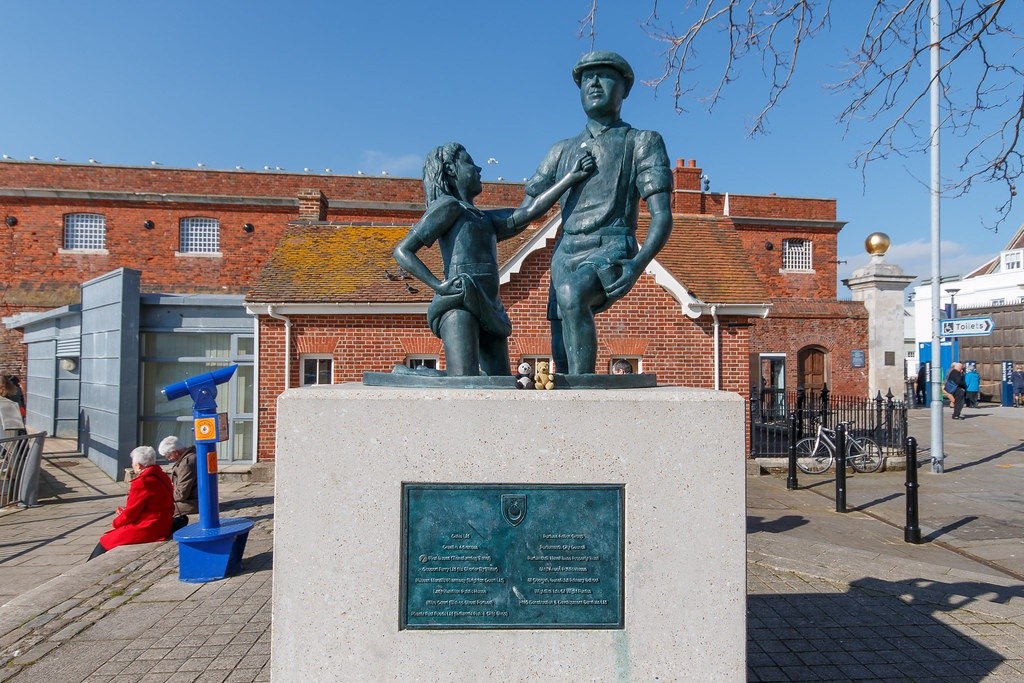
[391,52,674,376]
[1010,364,1024,408]
[612,360,632,375]
[0,375,29,460]
[86,436,199,563]
[916,362,980,420]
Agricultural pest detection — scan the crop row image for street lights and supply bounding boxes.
[944,288,960,362]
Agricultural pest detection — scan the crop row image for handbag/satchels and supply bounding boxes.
[944,379,958,394]
[168,515,189,537]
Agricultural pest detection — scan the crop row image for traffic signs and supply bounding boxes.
[939,316,996,338]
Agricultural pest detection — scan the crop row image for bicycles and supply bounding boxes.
[794,410,883,474]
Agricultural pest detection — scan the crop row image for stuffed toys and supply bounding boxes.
[515,361,554,390]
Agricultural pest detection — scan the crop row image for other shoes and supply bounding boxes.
[952,414,965,419]
